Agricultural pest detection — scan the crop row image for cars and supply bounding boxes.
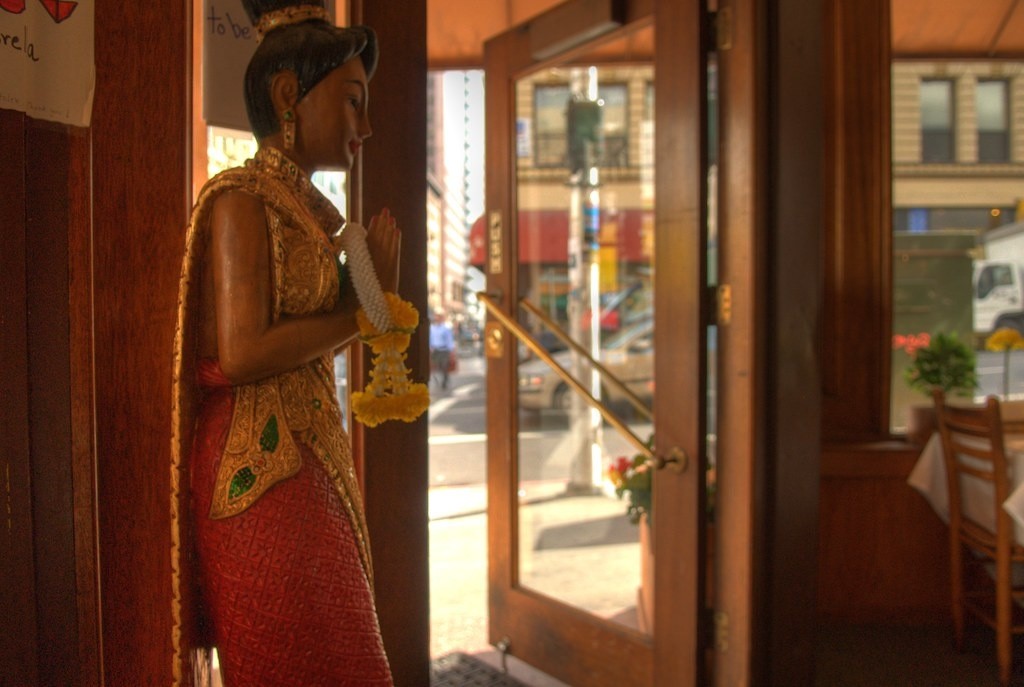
[518,286,653,427]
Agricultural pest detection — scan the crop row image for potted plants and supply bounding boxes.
[905,331,980,443]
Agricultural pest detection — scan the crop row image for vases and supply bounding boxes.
[636,510,714,636]
[998,401,1023,422]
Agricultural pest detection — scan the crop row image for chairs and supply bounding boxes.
[933,386,1023,686]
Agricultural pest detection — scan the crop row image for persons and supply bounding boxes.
[430,315,455,393]
[173,1,428,687]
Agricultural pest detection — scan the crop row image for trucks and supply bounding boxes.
[968,222,1024,354]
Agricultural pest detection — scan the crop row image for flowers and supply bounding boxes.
[351,293,432,428]
[610,433,716,522]
[986,325,1024,401]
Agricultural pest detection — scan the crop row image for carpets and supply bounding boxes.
[429,651,528,687]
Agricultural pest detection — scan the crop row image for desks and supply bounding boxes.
[907,429,1024,588]
